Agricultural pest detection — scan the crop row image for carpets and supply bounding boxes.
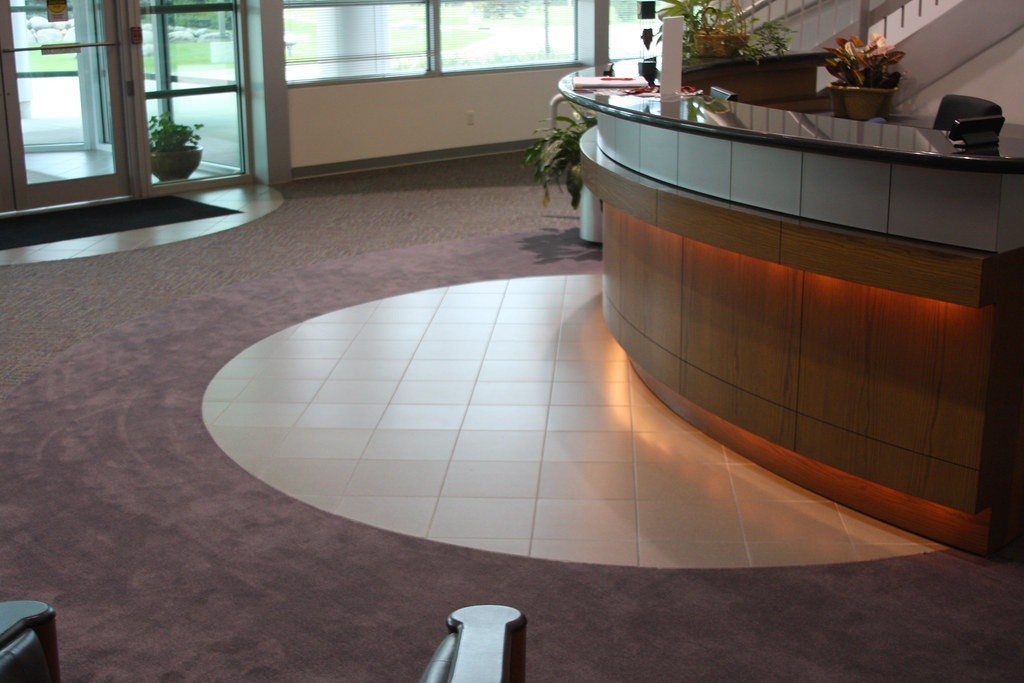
[0,195,245,250]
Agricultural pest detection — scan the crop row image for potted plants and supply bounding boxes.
[148,110,204,180]
[518,98,604,244]
[824,32,906,120]
[653,0,800,66]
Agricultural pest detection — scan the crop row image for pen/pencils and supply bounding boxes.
[601,77,636,81]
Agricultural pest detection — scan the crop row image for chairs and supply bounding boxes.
[934,95,1003,131]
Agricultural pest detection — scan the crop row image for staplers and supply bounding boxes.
[603,63,615,74]
